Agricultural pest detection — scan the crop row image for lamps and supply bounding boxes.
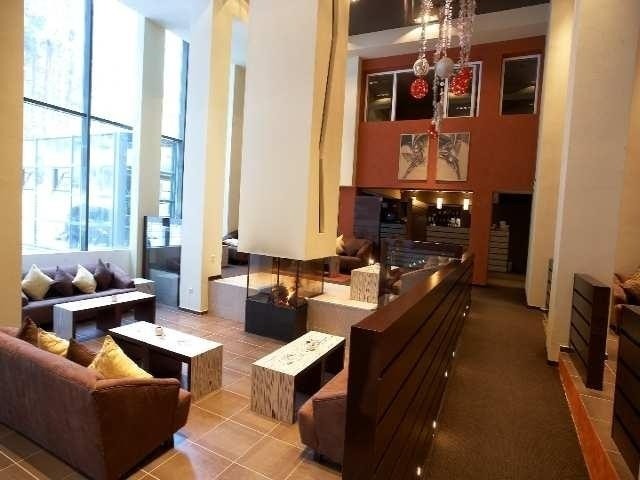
[435,193,445,209]
[411,0,478,135]
[462,192,470,211]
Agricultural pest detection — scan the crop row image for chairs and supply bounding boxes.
[294,368,345,463]
[342,238,373,272]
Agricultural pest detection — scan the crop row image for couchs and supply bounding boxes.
[0,327,191,480]
[19,262,135,329]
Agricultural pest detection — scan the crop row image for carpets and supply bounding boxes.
[266,266,350,287]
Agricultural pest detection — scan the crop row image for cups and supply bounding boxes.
[305,341,312,351]
[155,326,165,338]
[111,294,117,302]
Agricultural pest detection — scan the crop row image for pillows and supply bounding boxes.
[16,315,154,381]
[20,258,115,300]
[223,238,238,247]
[614,265,639,304]
